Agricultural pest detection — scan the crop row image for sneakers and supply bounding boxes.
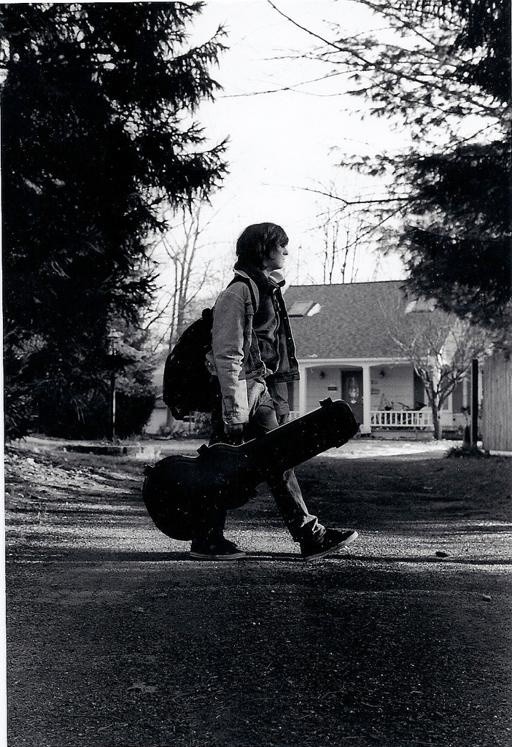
[299,528,358,561]
[190,540,247,559]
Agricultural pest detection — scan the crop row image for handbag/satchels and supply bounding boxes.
[162,276,257,414]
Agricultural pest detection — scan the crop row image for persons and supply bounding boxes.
[190,224,359,561]
